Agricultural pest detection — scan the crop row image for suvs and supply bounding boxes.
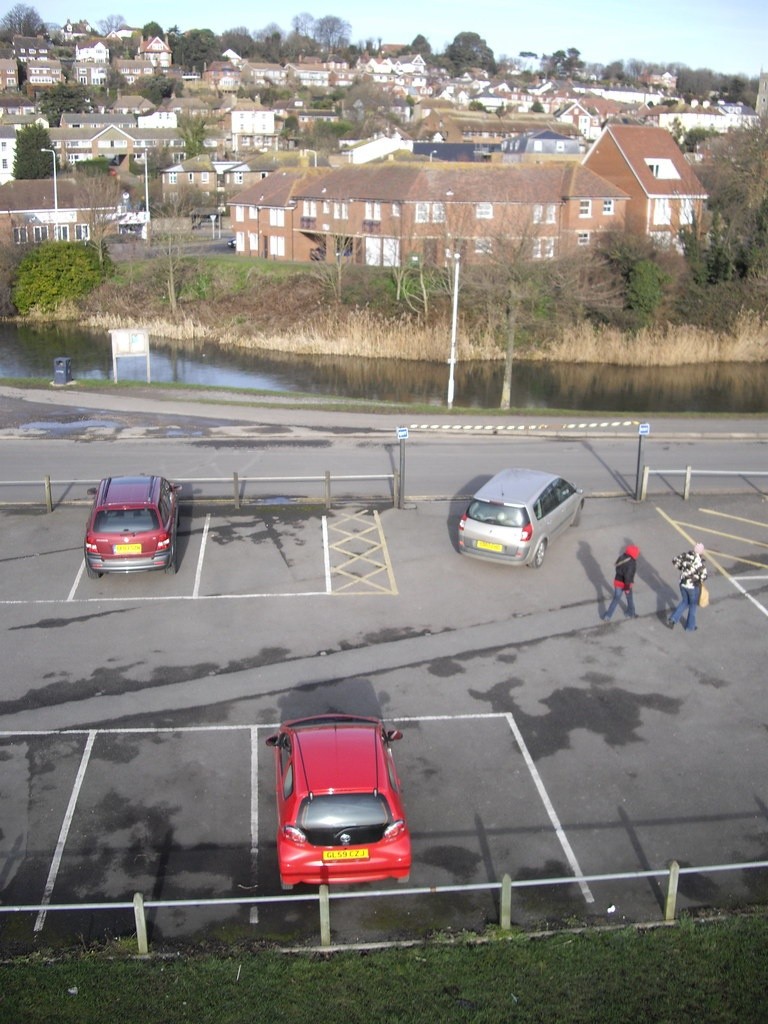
[84,474,182,577]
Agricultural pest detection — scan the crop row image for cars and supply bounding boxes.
[457,467,584,568]
[227,237,237,248]
[266,714,412,889]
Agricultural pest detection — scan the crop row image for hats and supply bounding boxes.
[694,542,704,554]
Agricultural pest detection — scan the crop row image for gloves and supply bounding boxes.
[625,590,630,594]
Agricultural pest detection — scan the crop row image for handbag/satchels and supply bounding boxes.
[698,583,709,608]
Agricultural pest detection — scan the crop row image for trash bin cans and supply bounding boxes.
[54,357,71,384]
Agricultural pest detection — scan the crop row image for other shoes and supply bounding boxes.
[686,626,697,632]
[669,618,674,629]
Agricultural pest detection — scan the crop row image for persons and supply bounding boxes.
[669,542,707,631]
[603,545,640,620]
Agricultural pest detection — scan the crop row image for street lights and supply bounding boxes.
[41,148,59,242]
[447,253,461,408]
[305,149,317,167]
[144,148,148,243]
[430,150,437,161]
